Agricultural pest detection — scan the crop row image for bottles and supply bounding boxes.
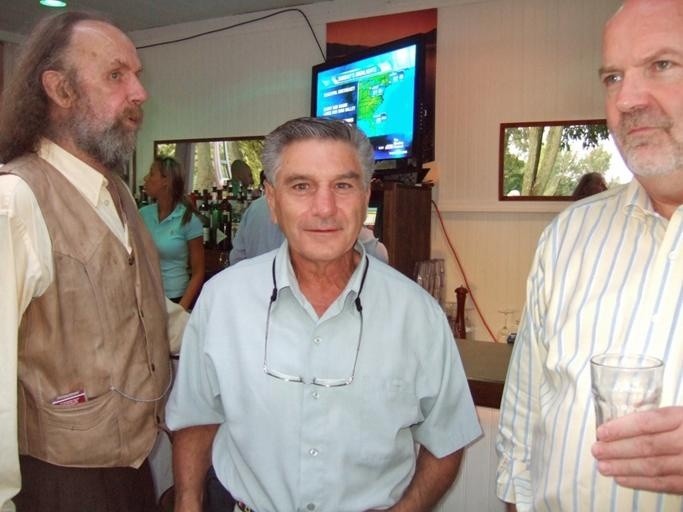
[134,182,156,209]
[190,181,263,253]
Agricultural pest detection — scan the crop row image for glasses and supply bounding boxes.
[262,292,364,387]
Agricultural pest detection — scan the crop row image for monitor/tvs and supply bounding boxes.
[311,33,423,161]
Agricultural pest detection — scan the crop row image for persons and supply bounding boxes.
[166,116,485,512]
[230,170,286,266]
[0,10,193,512]
[493,0,683,512]
[137,155,206,311]
[571,171,609,198]
[231,160,254,188]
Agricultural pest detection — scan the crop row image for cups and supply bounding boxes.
[590,352,666,441]
